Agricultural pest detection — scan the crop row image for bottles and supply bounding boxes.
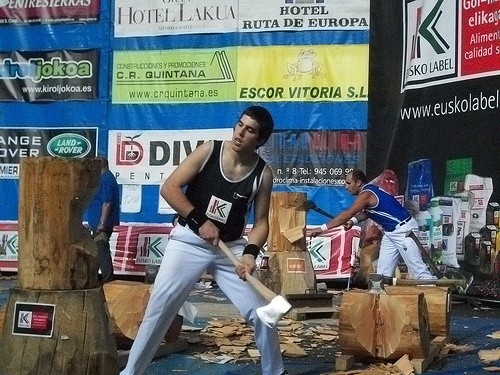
[427,201,442,263]
[416,204,432,256]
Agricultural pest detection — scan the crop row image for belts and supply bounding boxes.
[400,216,412,226]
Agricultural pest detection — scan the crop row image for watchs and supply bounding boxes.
[99,221,106,225]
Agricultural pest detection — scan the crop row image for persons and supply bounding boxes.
[90,158,120,285]
[117,105,290,375]
[309,169,442,287]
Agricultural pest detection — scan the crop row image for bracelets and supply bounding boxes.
[350,216,358,225]
[243,245,259,259]
[321,223,328,233]
